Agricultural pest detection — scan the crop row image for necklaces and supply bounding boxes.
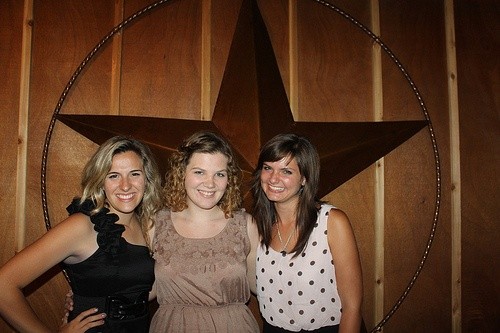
[272,212,294,253]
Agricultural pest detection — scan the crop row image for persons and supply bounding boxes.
[140,134,261,333]
[0,136,159,333]
[241,134,369,333]
